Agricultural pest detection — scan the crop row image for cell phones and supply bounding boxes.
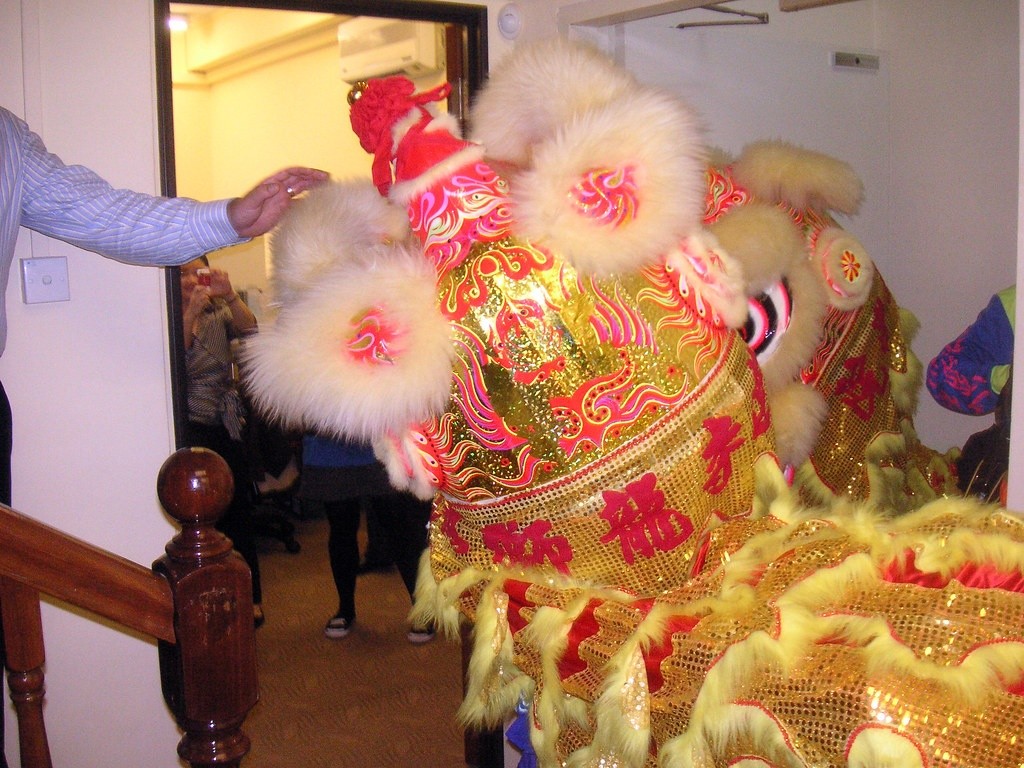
[196,269,210,292]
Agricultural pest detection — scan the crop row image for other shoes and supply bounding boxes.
[254,605,266,628]
[325,613,356,636]
[408,626,435,643]
[358,557,393,573]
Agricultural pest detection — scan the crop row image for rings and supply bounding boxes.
[287,188,296,199]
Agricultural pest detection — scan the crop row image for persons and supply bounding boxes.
[0,102,330,512]
[178,250,266,626]
[303,428,438,644]
[927,278,1017,507]
